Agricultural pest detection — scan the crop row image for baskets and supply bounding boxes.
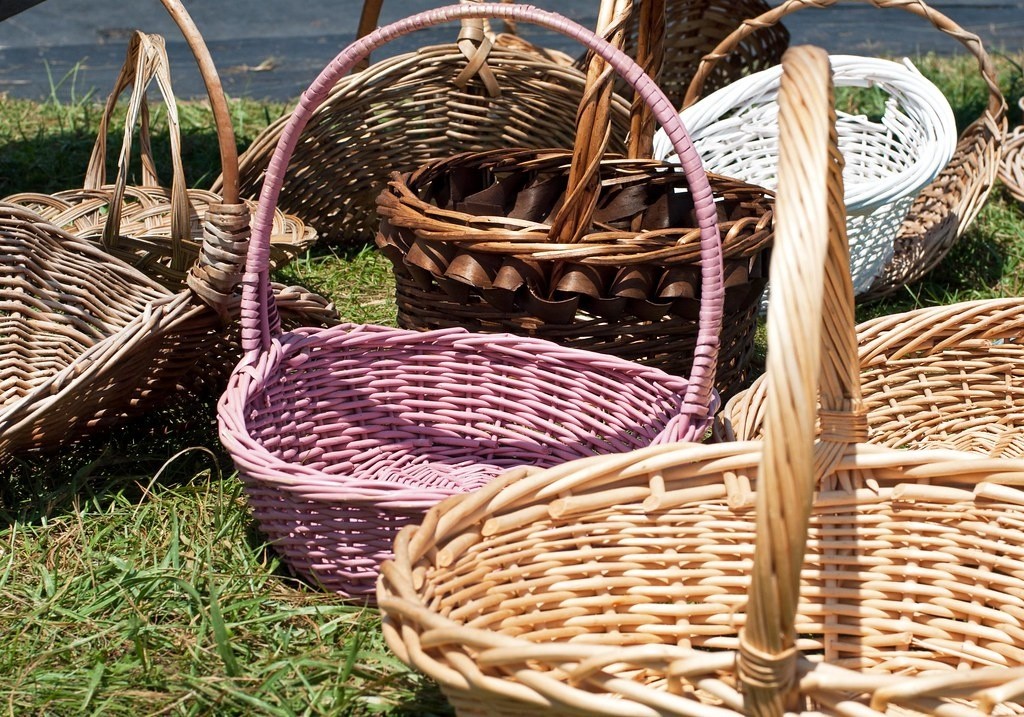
[372,41,1023,717]
[0,29,319,291]
[712,299,1024,461]
[654,53,956,296]
[208,0,632,250]
[571,0,789,131]
[680,0,1010,303]
[217,1,727,600]
[380,0,776,404]
[999,100,1024,204]
[1,0,342,463]
